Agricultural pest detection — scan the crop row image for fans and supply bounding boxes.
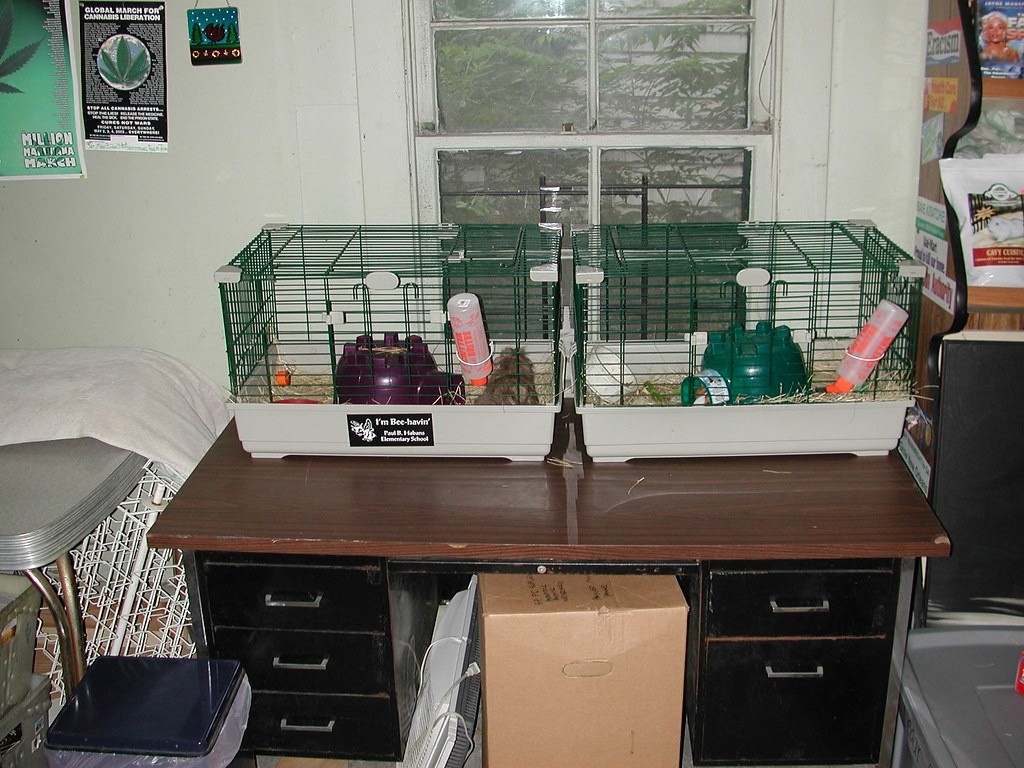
[394,573,482,768]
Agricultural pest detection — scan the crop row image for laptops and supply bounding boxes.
[45,655,243,750]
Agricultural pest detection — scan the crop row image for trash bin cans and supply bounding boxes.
[897,625,1024,768]
[42,656,258,768]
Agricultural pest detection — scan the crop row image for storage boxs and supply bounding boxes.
[0,573,43,720]
[898,625,1024,768]
[0,672,54,768]
[478,574,689,768]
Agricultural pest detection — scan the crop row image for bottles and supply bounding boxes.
[827,298,908,393]
[447,293,492,387]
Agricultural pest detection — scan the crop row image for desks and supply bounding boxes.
[146,398,954,768]
[0,437,194,702]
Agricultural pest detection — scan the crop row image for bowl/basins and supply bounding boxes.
[586,346,638,403]
